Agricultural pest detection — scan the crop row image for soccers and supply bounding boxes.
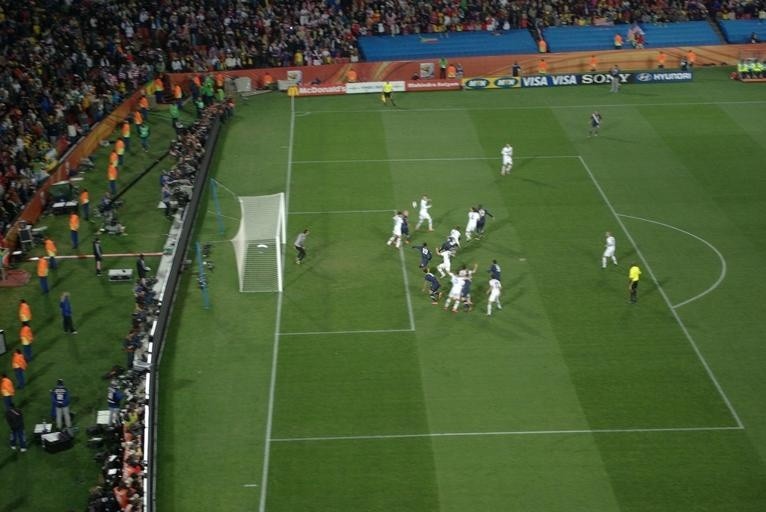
[411,201,419,207]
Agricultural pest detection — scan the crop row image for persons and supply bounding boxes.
[601,231,619,268]
[294,229,311,264]
[238,0,766,95]
[386,194,504,317]
[1,0,238,510]
[626,261,643,304]
[382,79,398,106]
[500,144,514,176]
[587,110,603,138]
